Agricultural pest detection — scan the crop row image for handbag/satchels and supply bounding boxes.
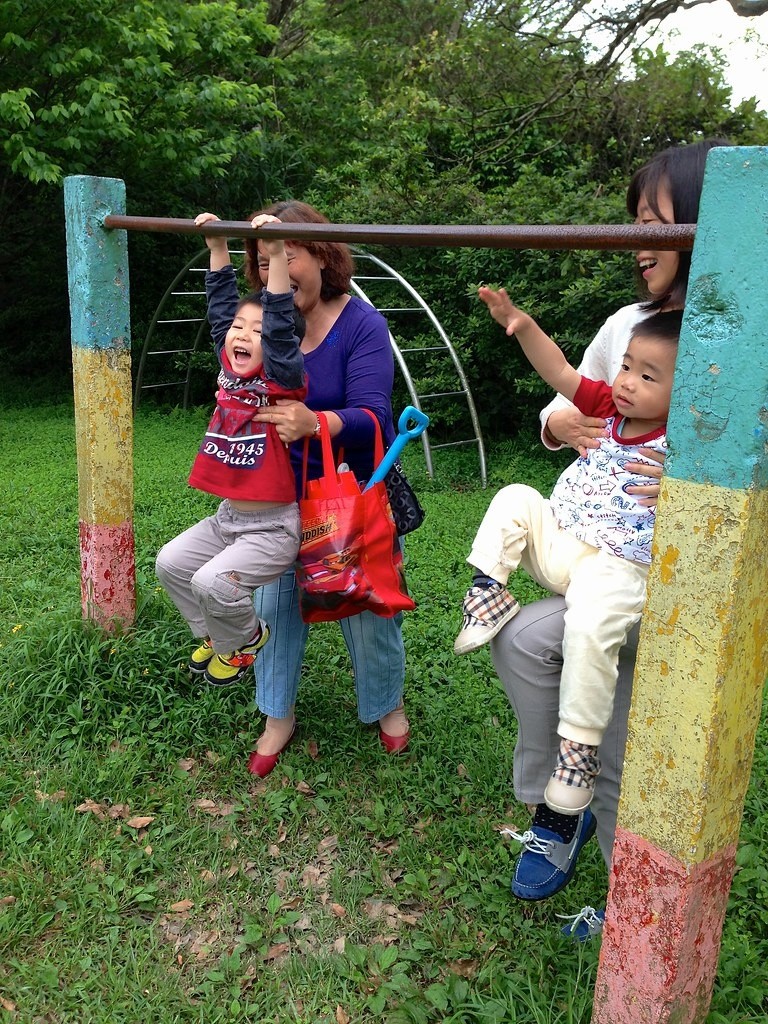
[296,407,415,624]
[363,459,425,536]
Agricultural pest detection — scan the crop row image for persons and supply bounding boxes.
[488,140,728,945]
[156,212,309,687]
[455,286,686,813]
[214,200,409,774]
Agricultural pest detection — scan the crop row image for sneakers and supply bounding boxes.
[189,618,271,686]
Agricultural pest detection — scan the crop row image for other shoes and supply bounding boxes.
[513,803,598,900]
[452,583,520,654]
[544,738,600,814]
[556,906,606,942]
[381,714,410,756]
[247,720,297,778]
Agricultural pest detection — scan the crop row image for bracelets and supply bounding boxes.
[312,416,321,438]
